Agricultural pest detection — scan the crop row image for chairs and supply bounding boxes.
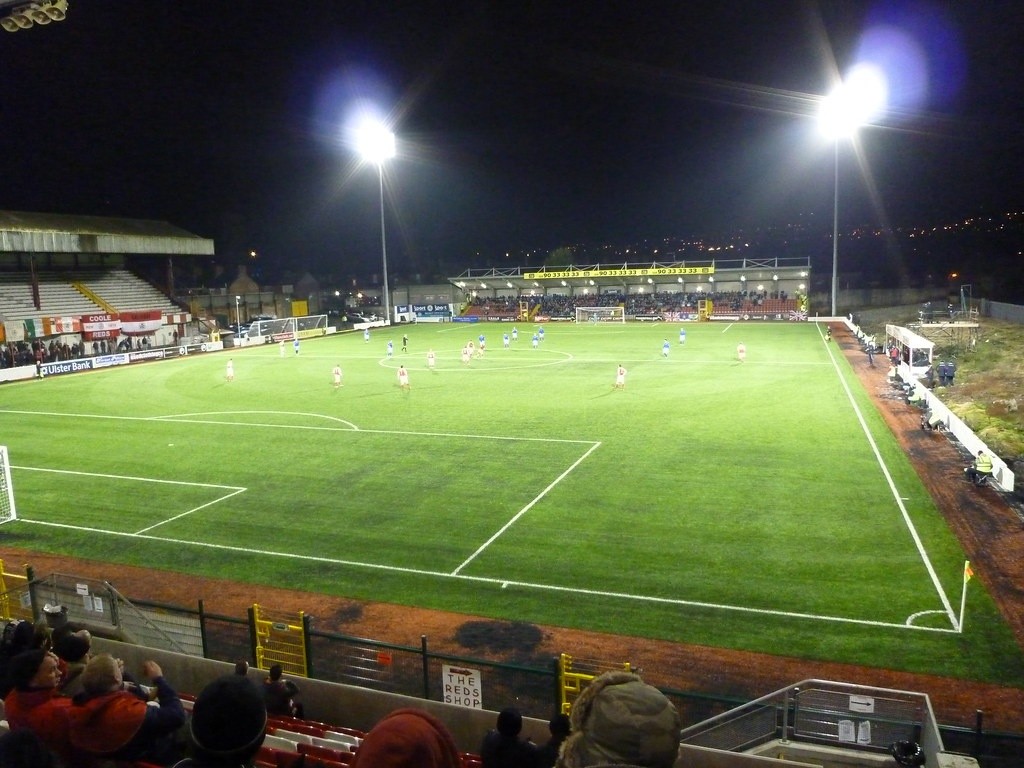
[465,296,796,319]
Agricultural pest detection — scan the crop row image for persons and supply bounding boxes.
[351,673,680,768]
[921,404,933,430]
[401,334,408,352]
[532,326,544,348]
[341,315,347,326]
[889,739,926,768]
[737,343,745,360]
[503,327,518,347]
[333,365,342,385]
[0,620,305,768]
[387,340,392,356]
[427,349,434,367]
[226,359,233,379]
[925,302,955,323]
[463,334,485,366]
[279,338,299,355]
[867,339,955,388]
[680,327,686,344]
[398,366,410,391]
[474,289,788,325]
[663,339,670,357]
[848,312,861,325]
[827,326,832,341]
[0,330,178,369]
[365,332,369,341]
[966,450,993,483]
[615,364,627,389]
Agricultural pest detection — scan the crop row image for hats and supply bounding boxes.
[7,639,53,689]
[497,710,522,734]
[352,707,461,768]
[189,673,269,765]
[555,671,680,768]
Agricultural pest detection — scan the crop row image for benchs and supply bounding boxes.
[0,262,191,339]
[138,688,484,768]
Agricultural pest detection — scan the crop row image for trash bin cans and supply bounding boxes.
[894,740,921,768]
[42,606,69,628]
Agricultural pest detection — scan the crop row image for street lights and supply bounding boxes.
[350,115,396,322]
[820,64,883,316]
[235,295,243,339]
